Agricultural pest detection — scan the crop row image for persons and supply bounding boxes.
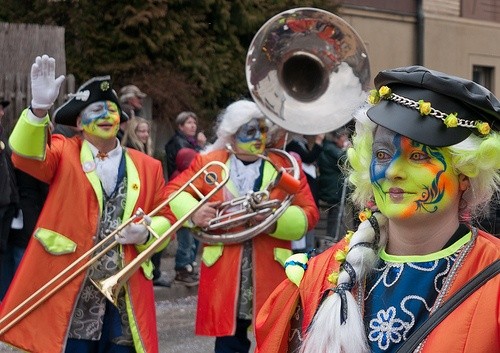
[154,100,321,353]
[0,86,500,301]
[254,66,500,353]
[0,55,178,353]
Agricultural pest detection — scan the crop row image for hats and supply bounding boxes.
[120,86,147,98]
[0,97,11,108]
[53,76,130,127]
[368,66,500,146]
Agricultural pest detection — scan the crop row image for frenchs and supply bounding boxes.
[190,8,372,246]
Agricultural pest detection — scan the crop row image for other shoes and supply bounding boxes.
[174,270,200,287]
[153,277,171,287]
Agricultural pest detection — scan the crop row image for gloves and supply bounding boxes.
[31,55,64,108]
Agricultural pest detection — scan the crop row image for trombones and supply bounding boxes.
[0,160,230,335]
